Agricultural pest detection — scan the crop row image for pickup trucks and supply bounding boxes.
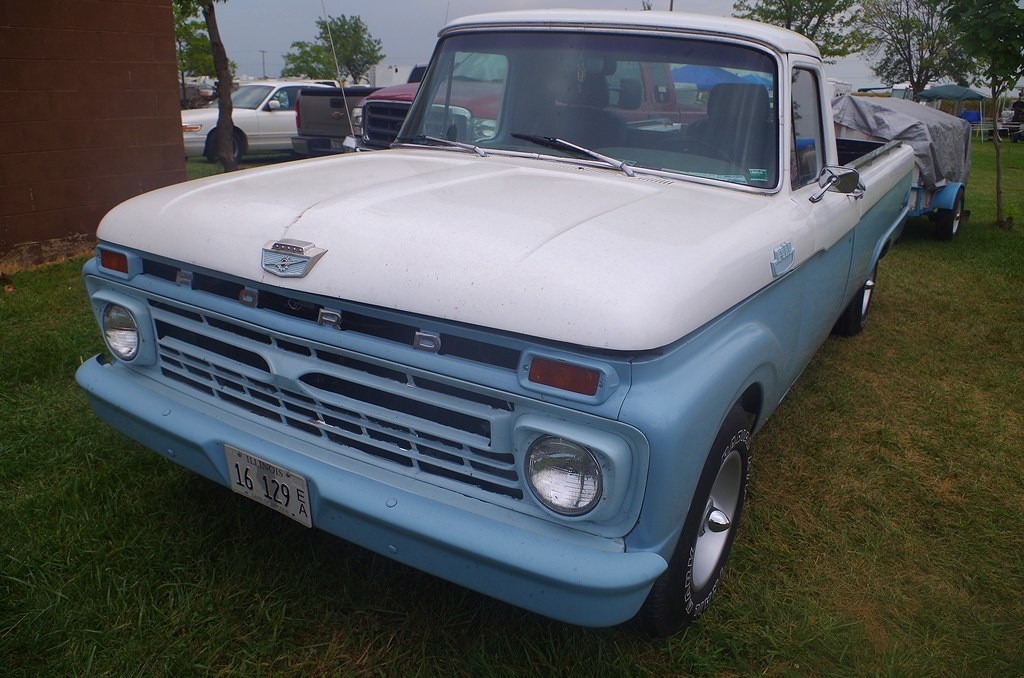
[75,12,918,632]
[293,64,426,157]
[354,39,680,153]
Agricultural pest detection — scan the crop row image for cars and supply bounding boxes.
[182,79,337,160]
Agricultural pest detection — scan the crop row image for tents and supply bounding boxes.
[663,65,774,106]
[914,83,1006,143]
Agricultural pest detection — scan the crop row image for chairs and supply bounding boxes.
[526,71,631,150]
[684,83,775,165]
